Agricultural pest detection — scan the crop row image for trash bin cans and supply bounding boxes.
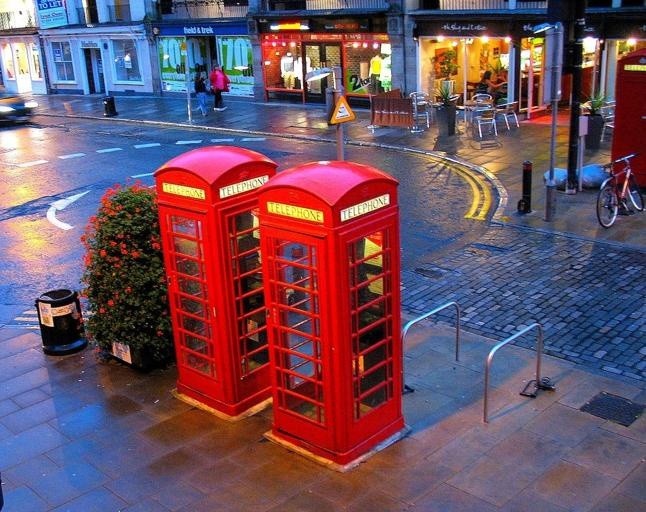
[37,290,89,355]
[102,97,119,116]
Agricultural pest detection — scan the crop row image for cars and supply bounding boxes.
[0,83,35,128]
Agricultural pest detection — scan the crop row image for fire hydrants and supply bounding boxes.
[63,101,74,115]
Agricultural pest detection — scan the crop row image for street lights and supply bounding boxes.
[532,21,559,223]
[304,67,344,162]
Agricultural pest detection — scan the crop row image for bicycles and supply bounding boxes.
[595,151,645,229]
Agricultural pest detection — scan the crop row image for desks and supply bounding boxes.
[464,100,495,128]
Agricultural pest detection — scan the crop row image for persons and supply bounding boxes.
[477,71,507,94]
[194,73,208,116]
[367,54,391,92]
[280,51,311,90]
[210,63,230,112]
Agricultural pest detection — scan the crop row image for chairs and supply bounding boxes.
[471,94,520,138]
[579,97,615,142]
[409,91,460,128]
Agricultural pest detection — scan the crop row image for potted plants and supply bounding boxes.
[581,88,612,150]
[432,80,456,136]
